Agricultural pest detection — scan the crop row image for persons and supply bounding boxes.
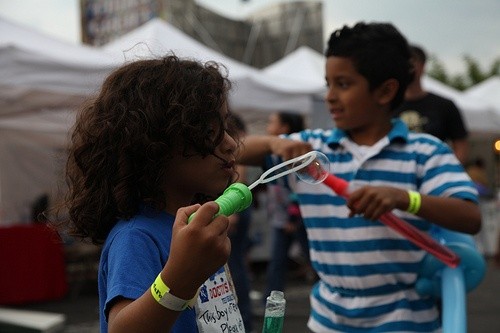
[402,45,470,143]
[260,111,314,301]
[64,56,245,333]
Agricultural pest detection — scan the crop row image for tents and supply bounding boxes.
[0,16,500,224]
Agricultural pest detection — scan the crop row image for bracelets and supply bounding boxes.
[408,190,420,215]
[150,275,196,312]
[232,20,482,333]
